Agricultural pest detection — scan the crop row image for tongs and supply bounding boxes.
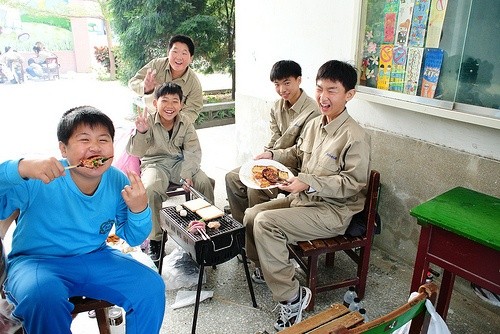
[180,179,212,204]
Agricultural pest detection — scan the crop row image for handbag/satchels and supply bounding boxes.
[113,128,140,182]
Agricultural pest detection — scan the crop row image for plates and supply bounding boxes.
[239,159,295,189]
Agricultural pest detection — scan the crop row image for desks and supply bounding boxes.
[409,185,500,334]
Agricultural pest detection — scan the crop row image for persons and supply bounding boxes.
[243,60,372,331]
[0,105,166,334]
[125,82,215,268]
[128,35,203,124]
[0,33,60,84]
[225,60,322,264]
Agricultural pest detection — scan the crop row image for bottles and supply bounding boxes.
[349,298,362,311]
[343,286,358,308]
[359,309,369,323]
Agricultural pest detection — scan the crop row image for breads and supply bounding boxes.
[196,205,225,222]
[252,165,289,187]
[182,198,211,212]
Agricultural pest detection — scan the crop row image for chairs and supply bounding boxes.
[276,282,438,334]
[0,210,115,334]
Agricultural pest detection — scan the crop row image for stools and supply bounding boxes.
[166,184,190,202]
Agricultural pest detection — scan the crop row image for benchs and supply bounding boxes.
[286,170,380,311]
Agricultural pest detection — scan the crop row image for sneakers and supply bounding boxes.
[251,267,266,284]
[271,286,312,331]
[237,247,252,263]
[147,227,167,262]
